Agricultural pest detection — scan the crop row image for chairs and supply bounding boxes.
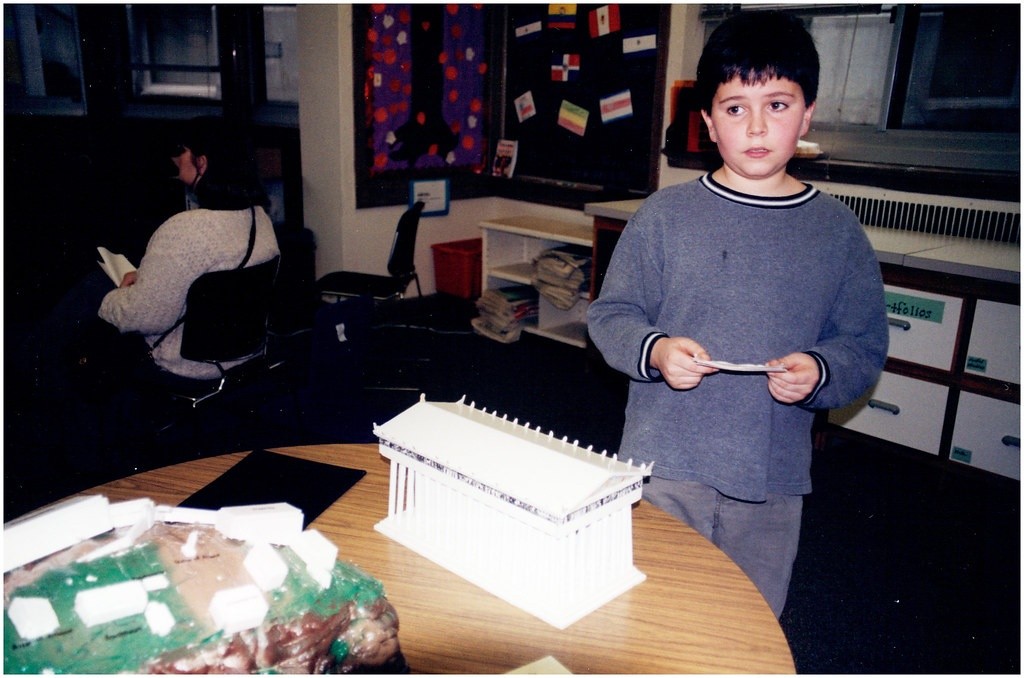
[93,256,283,471]
[312,201,435,391]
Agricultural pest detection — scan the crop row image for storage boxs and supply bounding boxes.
[431,237,483,299]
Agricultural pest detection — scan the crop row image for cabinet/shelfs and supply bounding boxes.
[479,216,594,349]
[816,263,1021,483]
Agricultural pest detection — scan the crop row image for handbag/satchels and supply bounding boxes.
[81,330,156,398]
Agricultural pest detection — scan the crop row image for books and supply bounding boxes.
[533,246,588,311]
[471,287,541,334]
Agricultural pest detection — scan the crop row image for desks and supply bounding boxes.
[3,442,798,673]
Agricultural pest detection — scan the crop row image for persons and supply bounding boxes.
[98,113,283,393]
[584,8,889,621]
